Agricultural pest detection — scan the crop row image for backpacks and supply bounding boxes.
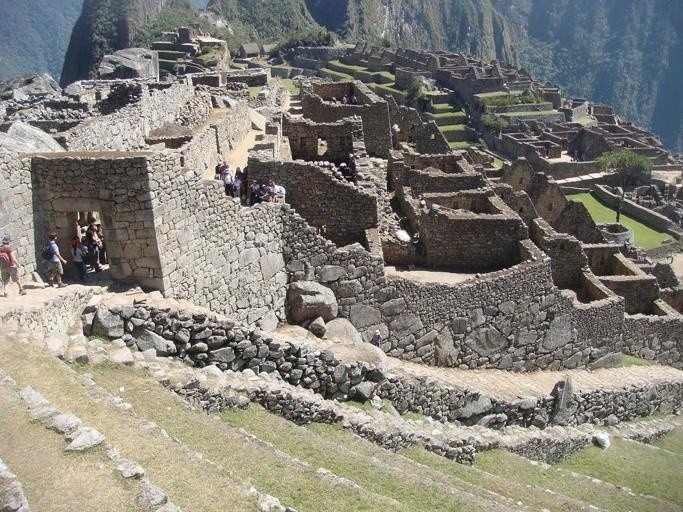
[0,252,10,268]
[42,241,56,261]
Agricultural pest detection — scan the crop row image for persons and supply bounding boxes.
[227,81,244,89]
[70,209,106,280]
[331,92,357,104]
[0,235,27,296]
[216,155,278,206]
[369,329,383,347]
[46,232,68,287]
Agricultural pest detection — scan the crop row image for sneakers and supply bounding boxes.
[57,281,68,287]
[49,279,54,287]
[19,288,27,296]
[4,291,9,298]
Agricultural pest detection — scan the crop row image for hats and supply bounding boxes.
[218,160,231,173]
[1,235,11,243]
[49,232,59,238]
